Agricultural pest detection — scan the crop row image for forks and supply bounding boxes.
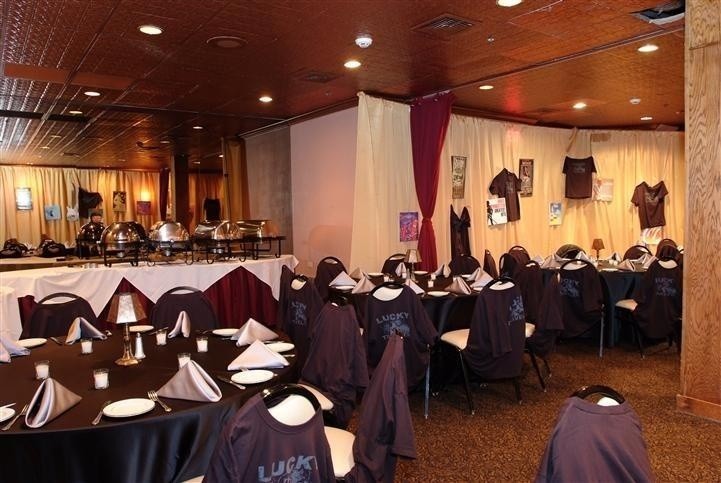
[2,405,29,430]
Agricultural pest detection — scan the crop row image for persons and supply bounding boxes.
[97,214,103,223]
[91,213,97,222]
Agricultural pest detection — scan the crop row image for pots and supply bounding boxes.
[76,219,279,262]
[383,272,461,288]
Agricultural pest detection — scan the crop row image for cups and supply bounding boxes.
[34,331,208,390]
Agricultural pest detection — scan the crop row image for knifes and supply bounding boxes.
[92,401,110,425]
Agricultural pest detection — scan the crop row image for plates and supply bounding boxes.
[1,325,295,422]
[602,268,618,272]
[330,271,483,297]
[642,267,649,271]
